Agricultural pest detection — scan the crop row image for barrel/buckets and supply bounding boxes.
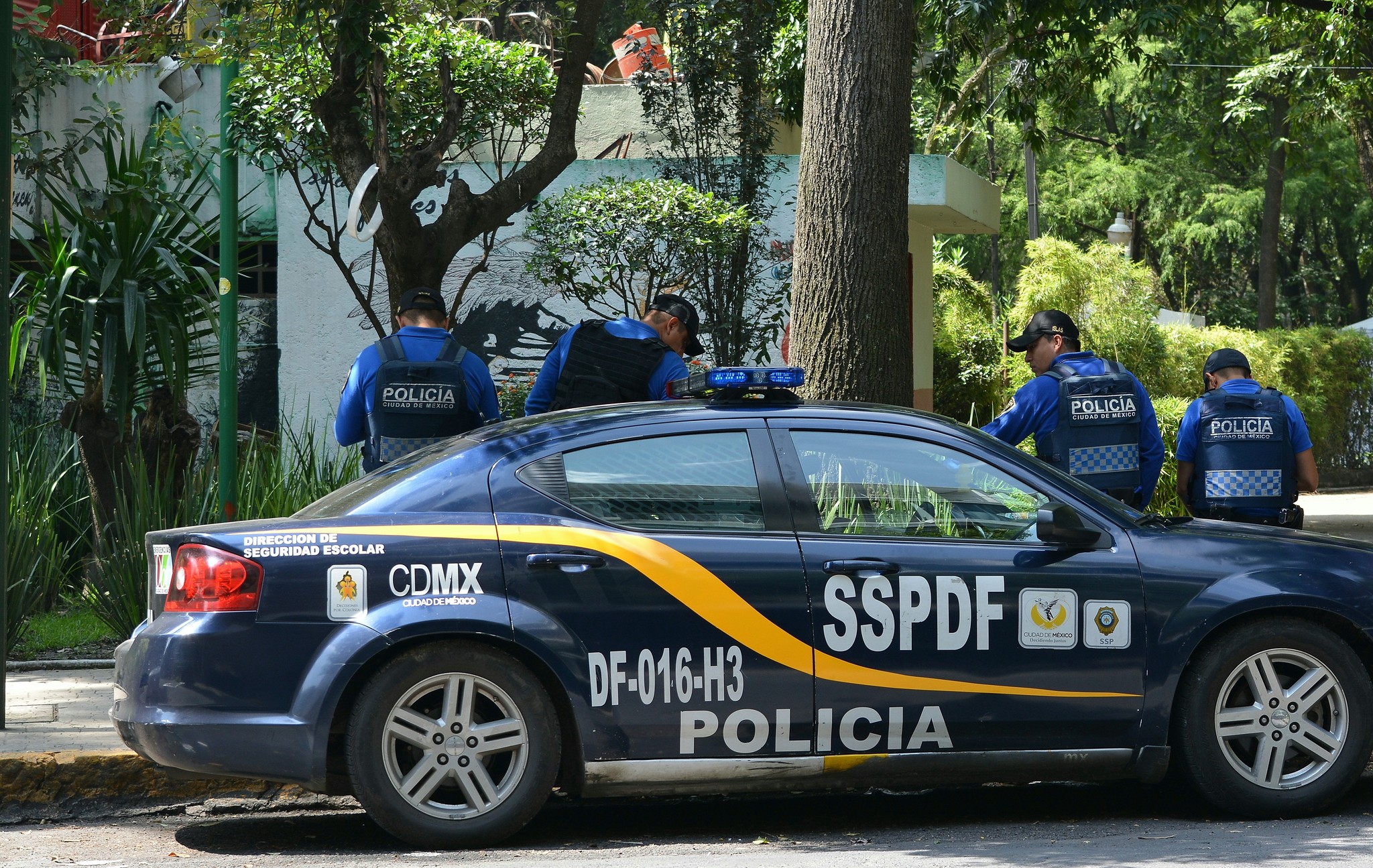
[553,57,626,85]
[612,23,679,83]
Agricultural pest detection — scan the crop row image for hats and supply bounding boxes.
[396,287,447,316]
[648,294,705,357]
[1006,308,1080,352]
[1203,348,1252,393]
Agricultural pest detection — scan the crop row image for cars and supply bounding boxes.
[107,367,1362,850]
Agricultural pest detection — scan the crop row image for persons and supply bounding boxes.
[525,293,708,418]
[1177,349,1319,525]
[975,310,1166,512]
[333,285,501,477]
